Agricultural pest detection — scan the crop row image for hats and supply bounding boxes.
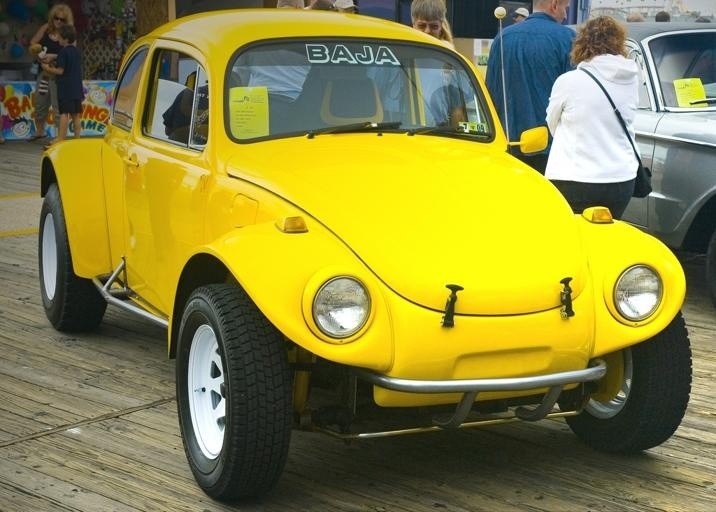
[509,7,530,17]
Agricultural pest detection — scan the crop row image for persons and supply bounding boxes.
[512,7,529,25]
[626,12,645,22]
[655,11,670,22]
[544,15,641,220]
[26,5,77,143]
[485,0,578,175]
[232,0,372,130]
[41,25,85,148]
[379,0,465,130]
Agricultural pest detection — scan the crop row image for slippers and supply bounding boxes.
[25,133,48,142]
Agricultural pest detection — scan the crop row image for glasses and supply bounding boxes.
[54,16,66,24]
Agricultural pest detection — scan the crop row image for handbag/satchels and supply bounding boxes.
[632,164,654,200]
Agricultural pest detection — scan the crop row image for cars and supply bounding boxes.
[519,14,716,314]
[32,6,697,509]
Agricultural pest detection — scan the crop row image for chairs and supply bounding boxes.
[318,78,383,130]
[169,71,242,147]
